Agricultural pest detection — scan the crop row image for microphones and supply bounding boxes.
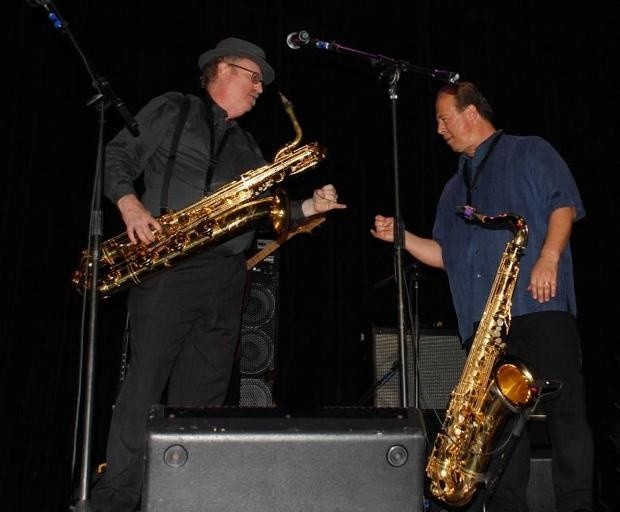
[286,29,311,51]
[91,73,139,130]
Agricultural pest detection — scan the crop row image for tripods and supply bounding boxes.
[353,96,411,408]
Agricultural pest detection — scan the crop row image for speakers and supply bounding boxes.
[370,324,468,409]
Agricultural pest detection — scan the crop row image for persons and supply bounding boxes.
[87,34,350,511]
[367,79,601,511]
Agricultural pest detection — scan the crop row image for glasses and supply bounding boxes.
[216,61,263,84]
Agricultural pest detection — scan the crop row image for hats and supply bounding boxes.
[199,37,275,85]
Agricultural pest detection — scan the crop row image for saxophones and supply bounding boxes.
[70,92,327,294]
[425,202,536,506]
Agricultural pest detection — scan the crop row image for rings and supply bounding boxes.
[544,281,550,285]
[334,195,338,202]
